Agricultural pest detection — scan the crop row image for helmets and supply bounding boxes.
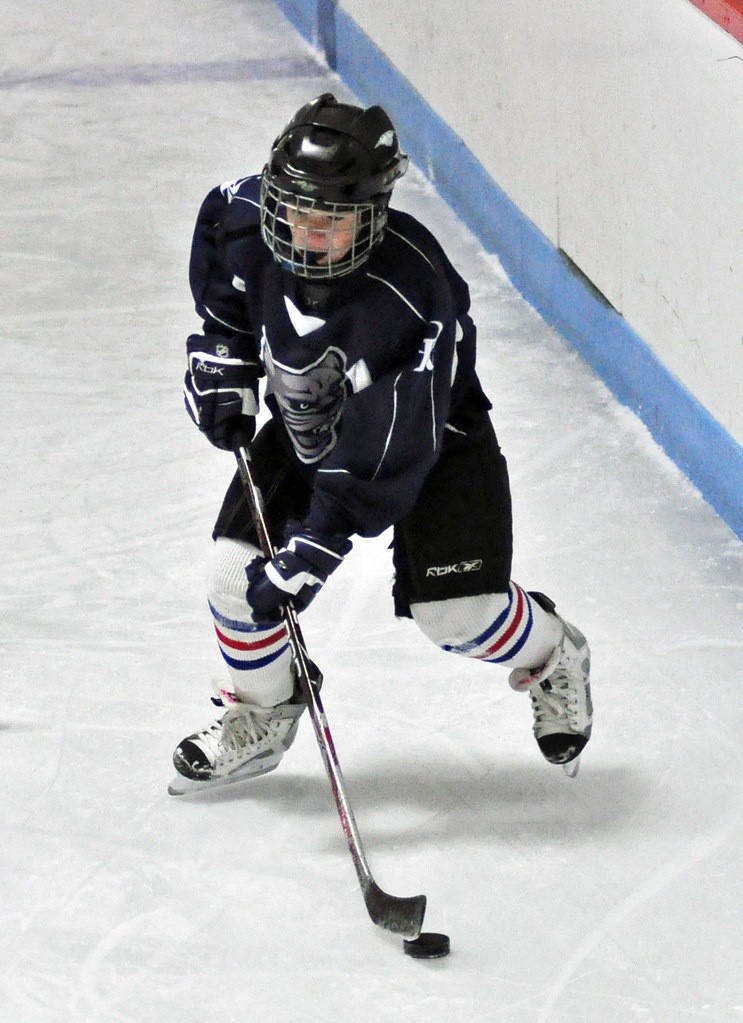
[259,94,409,278]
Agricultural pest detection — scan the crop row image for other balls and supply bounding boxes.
[403,932,450,959]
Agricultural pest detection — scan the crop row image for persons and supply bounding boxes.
[167,94,593,795]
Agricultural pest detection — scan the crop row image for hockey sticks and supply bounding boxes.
[233,444,426,941]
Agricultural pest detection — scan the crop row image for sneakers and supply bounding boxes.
[167,658,323,795]
[509,591,593,778]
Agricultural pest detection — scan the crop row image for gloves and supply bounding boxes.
[245,518,353,623]
[184,334,266,452]
